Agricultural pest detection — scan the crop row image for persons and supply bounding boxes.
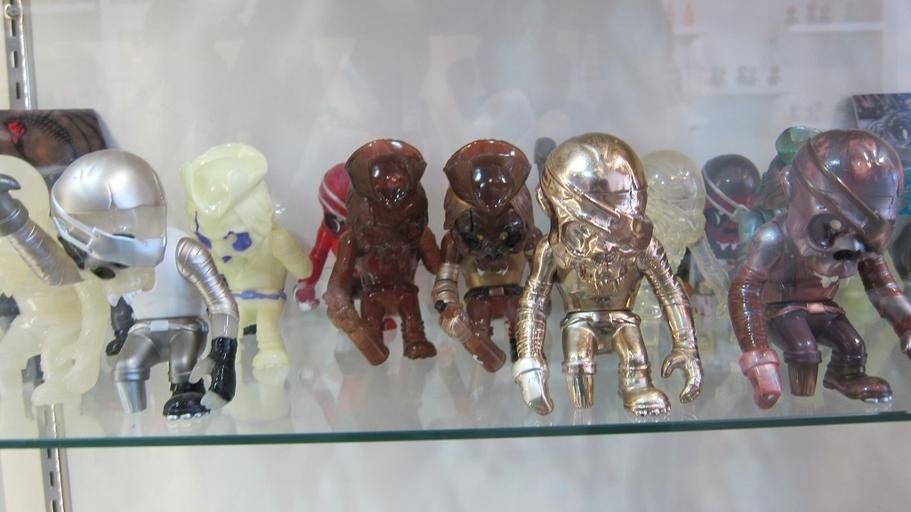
[294,138,543,372]
[513,133,704,417]
[630,110,911,408]
